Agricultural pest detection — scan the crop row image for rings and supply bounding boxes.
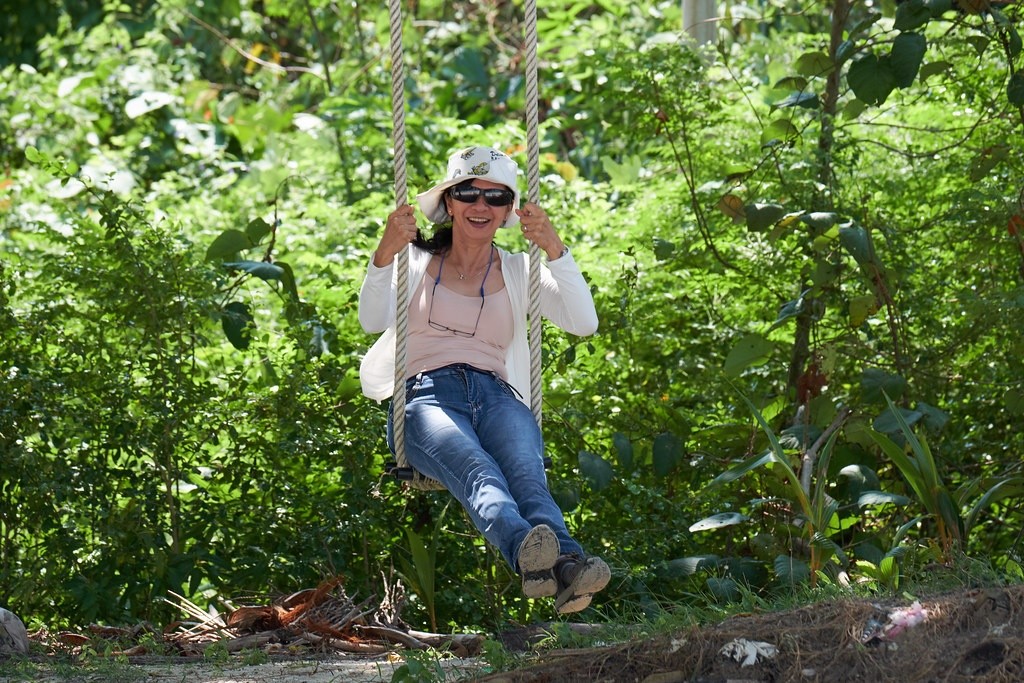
[524,226,527,232]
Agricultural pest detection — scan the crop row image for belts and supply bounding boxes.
[452,363,524,400]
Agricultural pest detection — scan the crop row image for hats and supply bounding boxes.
[416,144,521,230]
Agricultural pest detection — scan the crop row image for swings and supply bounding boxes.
[386,0,552,493]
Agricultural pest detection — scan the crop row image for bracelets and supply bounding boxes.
[548,247,568,262]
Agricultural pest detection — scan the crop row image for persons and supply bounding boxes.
[357,146,611,615]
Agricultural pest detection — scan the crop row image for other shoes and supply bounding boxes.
[518,524,563,602]
[554,551,612,612]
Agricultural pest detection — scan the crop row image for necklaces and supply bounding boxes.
[451,262,490,281]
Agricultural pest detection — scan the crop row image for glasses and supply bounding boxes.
[450,184,515,208]
[427,280,485,338]
